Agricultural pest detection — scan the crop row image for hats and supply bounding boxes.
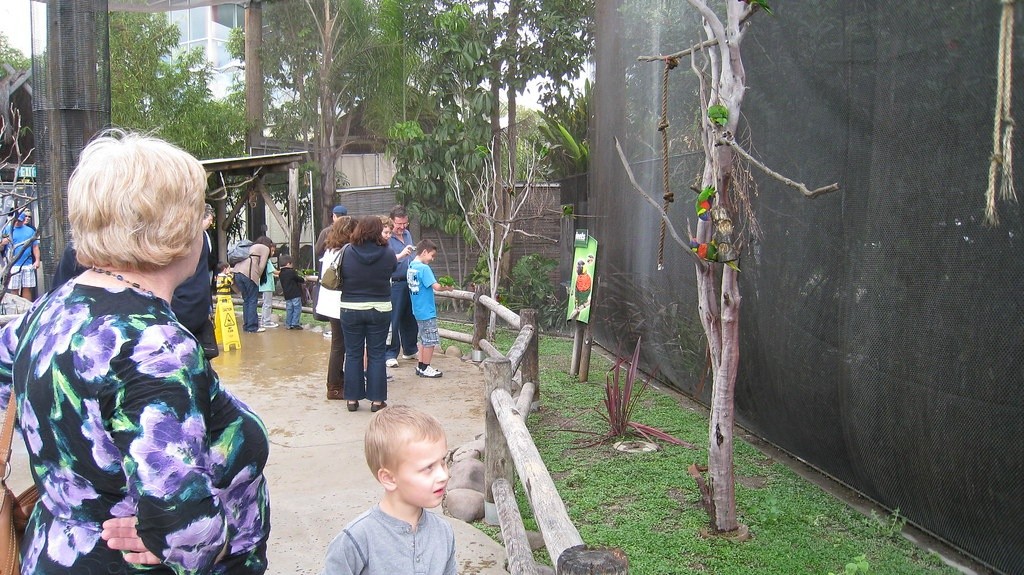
[331,206,348,217]
[13,211,25,221]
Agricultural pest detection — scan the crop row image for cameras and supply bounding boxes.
[409,246,417,252]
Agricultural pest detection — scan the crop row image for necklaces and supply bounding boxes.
[92,266,153,295]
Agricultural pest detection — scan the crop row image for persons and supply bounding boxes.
[323,405,458,575]
[312,206,419,401]
[170,202,230,362]
[0,132,270,575]
[340,214,397,413]
[0,208,40,302]
[407,238,454,377]
[230,236,306,330]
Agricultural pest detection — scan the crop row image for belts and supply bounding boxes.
[392,278,407,282]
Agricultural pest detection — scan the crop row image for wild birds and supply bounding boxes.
[496,293,512,309]
[689,237,741,273]
[575,260,592,320]
[696,183,717,221]
[295,269,307,281]
[561,205,576,220]
[439,276,462,290]
[505,185,516,198]
[301,268,320,275]
[738,0,776,18]
[468,281,476,293]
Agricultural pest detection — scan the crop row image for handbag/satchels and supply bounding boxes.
[1,478,39,575]
[320,244,349,291]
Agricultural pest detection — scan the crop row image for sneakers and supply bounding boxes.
[386,359,399,368]
[403,351,417,359]
[415,366,438,375]
[420,366,442,378]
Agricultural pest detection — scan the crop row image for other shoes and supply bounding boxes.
[371,401,387,412]
[249,327,266,333]
[327,384,345,399]
[363,368,394,382]
[324,331,332,337]
[347,401,359,411]
[262,322,278,328]
[286,325,302,330]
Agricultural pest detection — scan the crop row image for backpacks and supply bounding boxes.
[227,240,260,267]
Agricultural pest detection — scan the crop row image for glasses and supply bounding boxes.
[393,220,409,226]
